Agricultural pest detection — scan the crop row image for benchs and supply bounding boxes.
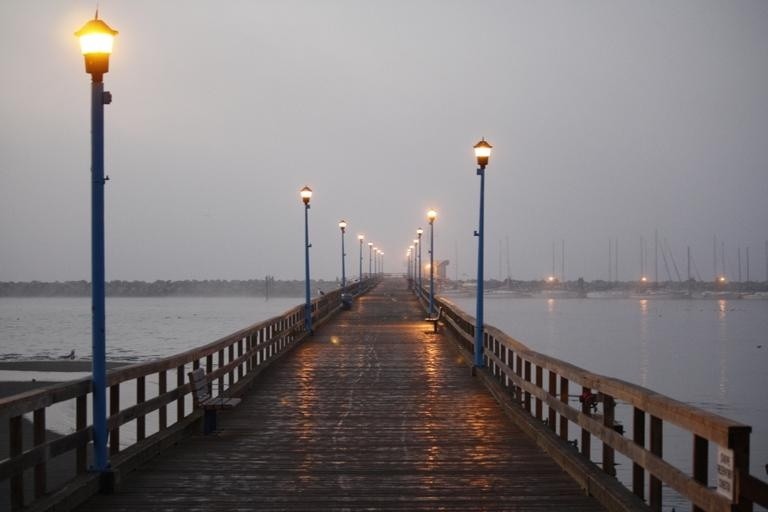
[187,367,244,436]
[425,306,444,333]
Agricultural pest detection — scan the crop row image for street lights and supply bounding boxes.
[472,137,493,370]
[339,219,385,296]
[75,4,119,474]
[299,184,313,334]
[406,209,438,313]
